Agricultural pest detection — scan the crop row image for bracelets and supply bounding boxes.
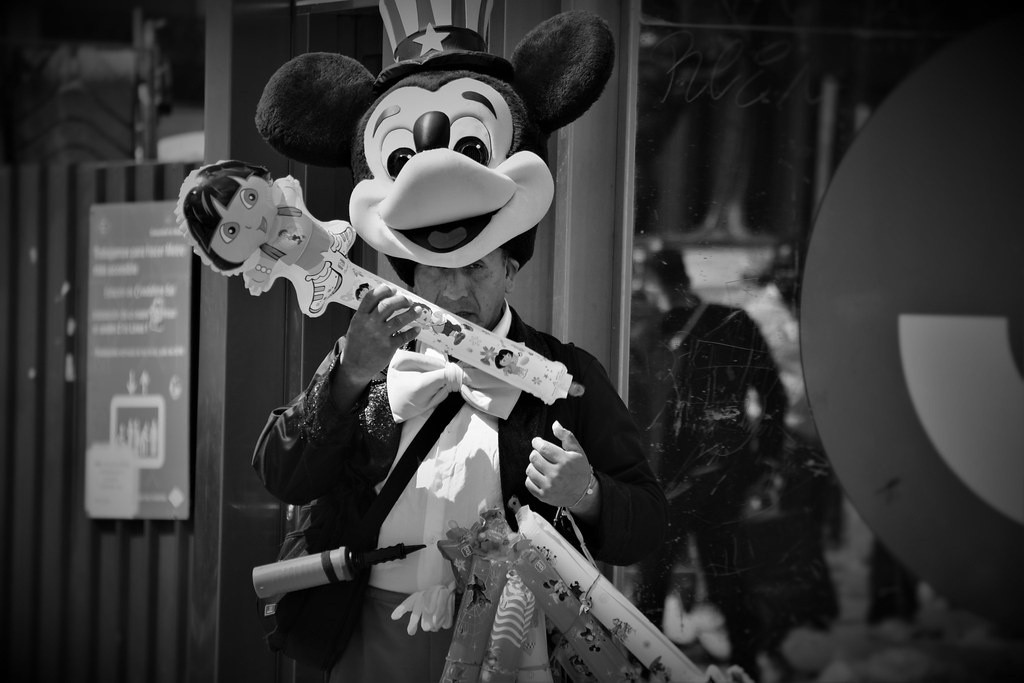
[567,466,594,509]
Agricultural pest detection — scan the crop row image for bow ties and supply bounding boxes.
[386,349,522,420]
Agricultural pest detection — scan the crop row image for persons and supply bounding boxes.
[252,156,669,683]
[616,229,931,676]
